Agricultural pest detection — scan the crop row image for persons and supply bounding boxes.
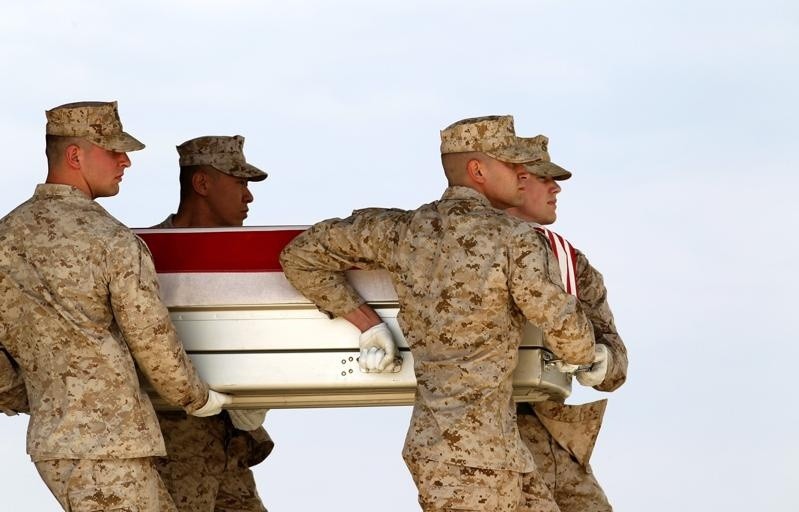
[512,134,630,511]
[154,134,275,510]
[0,96,234,512]
[277,113,597,510]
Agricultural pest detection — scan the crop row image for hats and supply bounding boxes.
[176,135,268,182]
[440,114,541,164]
[44,100,146,153]
[514,135,572,182]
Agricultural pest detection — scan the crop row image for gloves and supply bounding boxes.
[228,408,268,432]
[190,389,232,418]
[556,343,608,386]
[358,323,400,372]
